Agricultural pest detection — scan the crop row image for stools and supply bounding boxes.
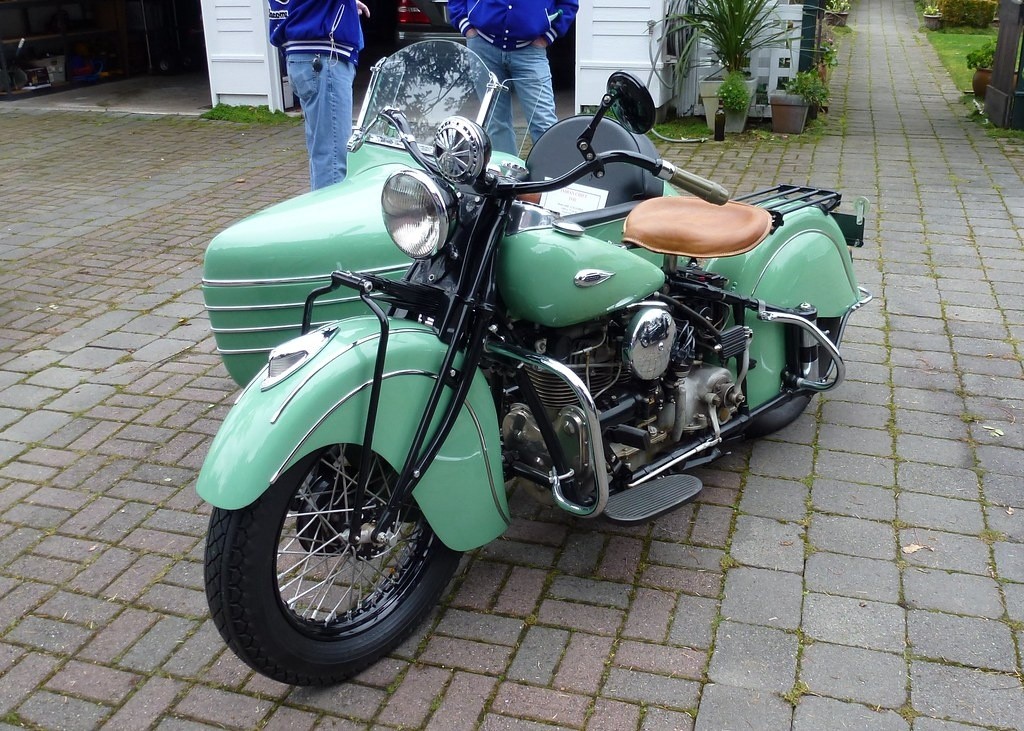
[621,192,772,280]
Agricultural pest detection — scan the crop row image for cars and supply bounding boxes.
[393,0,467,45]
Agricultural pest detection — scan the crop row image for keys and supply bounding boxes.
[313,53,322,83]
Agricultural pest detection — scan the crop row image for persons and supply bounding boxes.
[446,0,579,157]
[269,0,370,190]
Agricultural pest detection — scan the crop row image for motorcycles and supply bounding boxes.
[191,37,873,688]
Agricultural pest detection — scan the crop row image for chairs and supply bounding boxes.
[514,112,665,216]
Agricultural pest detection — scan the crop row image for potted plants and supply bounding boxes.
[768,67,831,134]
[923,2,943,29]
[967,39,1019,97]
[642,0,848,134]
[814,38,839,87]
[824,0,852,27]
